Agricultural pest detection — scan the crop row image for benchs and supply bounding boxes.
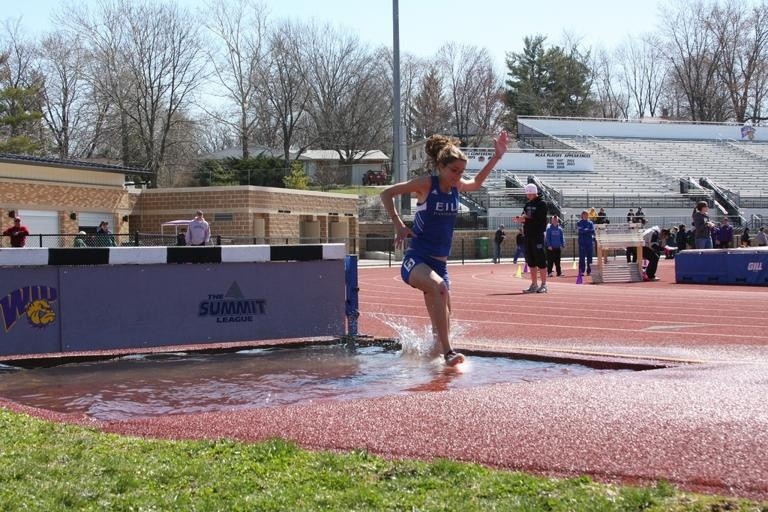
[463,139,768,208]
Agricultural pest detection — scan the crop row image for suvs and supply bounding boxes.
[361,169,387,186]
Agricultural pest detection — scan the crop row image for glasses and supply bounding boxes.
[446,165,465,176]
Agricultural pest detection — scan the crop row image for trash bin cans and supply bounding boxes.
[475,237,490,259]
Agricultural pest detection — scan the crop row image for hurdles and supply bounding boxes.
[0,243,360,356]
[589,222,646,283]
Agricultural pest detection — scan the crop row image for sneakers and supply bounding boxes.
[523,284,538,293]
[444,349,465,366]
[537,285,548,293]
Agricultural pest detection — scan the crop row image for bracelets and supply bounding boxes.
[391,213,400,221]
[493,154,501,161]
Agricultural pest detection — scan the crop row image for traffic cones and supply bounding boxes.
[515,257,583,285]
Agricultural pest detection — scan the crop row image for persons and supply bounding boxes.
[492,223,506,263]
[185,210,211,246]
[177,228,187,244]
[362,167,388,186]
[2,217,31,248]
[96,221,117,246]
[380,130,509,368]
[511,184,768,294]
[74,230,88,247]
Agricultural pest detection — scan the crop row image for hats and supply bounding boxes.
[524,183,538,195]
